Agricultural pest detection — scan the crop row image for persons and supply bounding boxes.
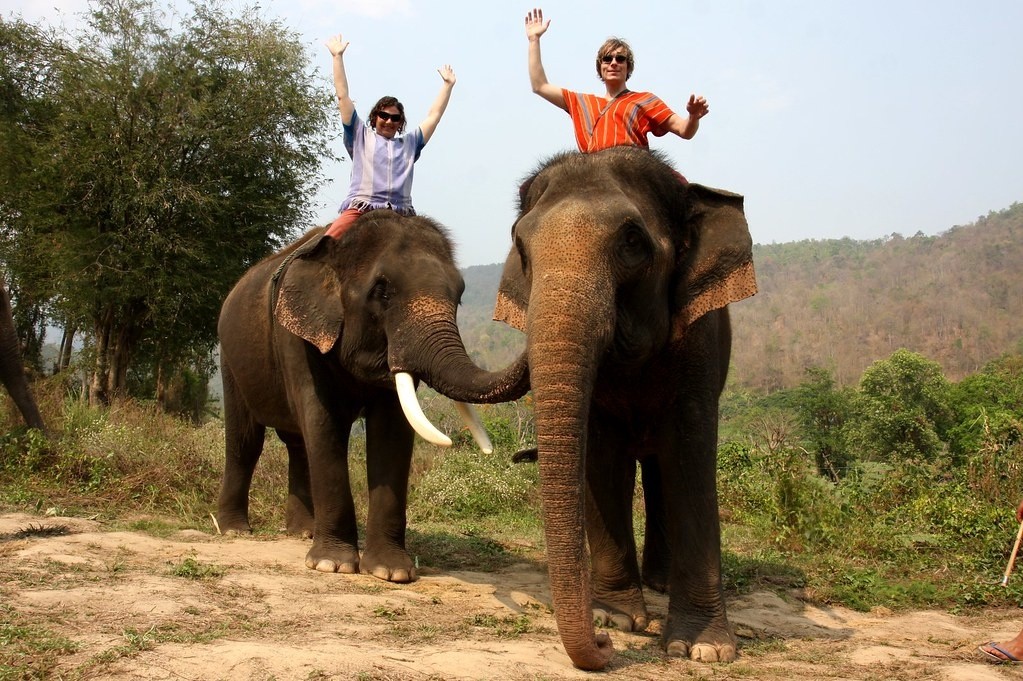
[525,8,709,155]
[323,34,456,240]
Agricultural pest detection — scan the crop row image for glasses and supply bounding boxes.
[376,110,401,122]
[600,55,628,64]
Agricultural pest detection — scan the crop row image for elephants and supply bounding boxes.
[216,208,531,583]
[489,140,764,672]
